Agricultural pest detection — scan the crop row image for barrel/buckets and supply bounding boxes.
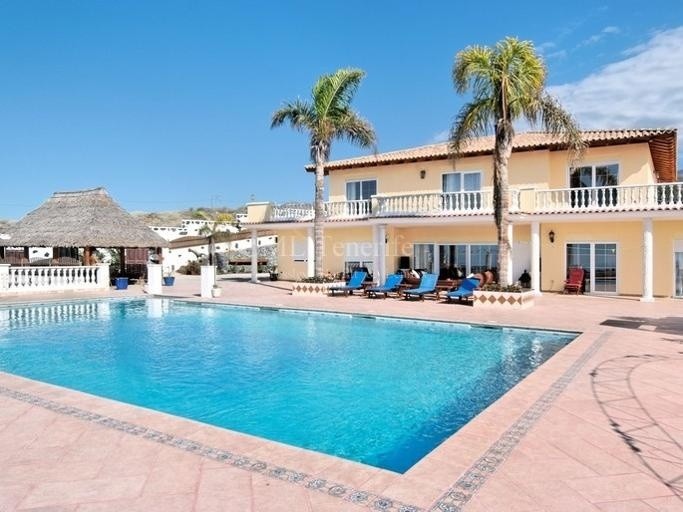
[115,278,129,288]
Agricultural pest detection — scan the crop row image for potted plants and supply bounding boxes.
[212,284,222,298]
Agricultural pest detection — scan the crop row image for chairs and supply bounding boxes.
[330,270,403,300]
[562,268,585,296]
[402,274,440,301]
[442,277,480,305]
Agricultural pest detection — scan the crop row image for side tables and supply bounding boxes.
[435,285,453,300]
[394,283,417,299]
